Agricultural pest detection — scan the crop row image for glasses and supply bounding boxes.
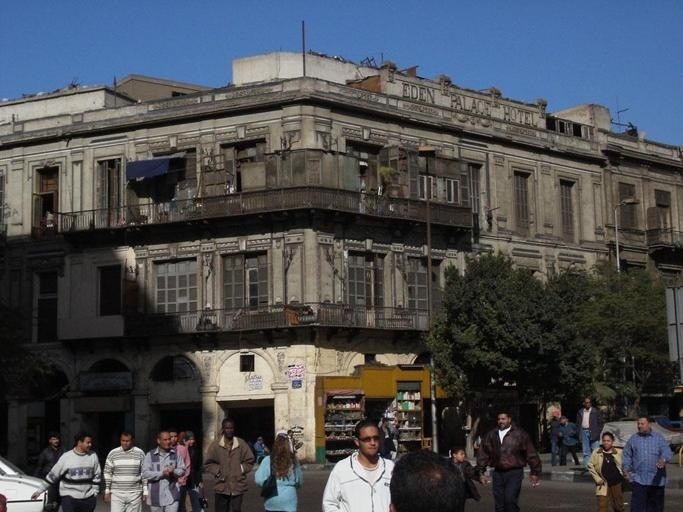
[360,435,380,442]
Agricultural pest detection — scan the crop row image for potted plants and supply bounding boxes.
[378,165,399,198]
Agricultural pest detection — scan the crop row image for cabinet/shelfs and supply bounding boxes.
[325,389,365,462]
[396,380,422,439]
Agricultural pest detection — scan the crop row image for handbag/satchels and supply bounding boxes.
[260,473,278,498]
[199,496,209,509]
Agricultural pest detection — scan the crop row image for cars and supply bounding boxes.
[0,455,59,512]
[599,415,681,454]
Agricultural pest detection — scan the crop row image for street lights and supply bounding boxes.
[416,144,443,454]
[614,196,641,418]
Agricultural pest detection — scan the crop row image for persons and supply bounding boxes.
[42,210,54,239]
[226,179,234,193]
